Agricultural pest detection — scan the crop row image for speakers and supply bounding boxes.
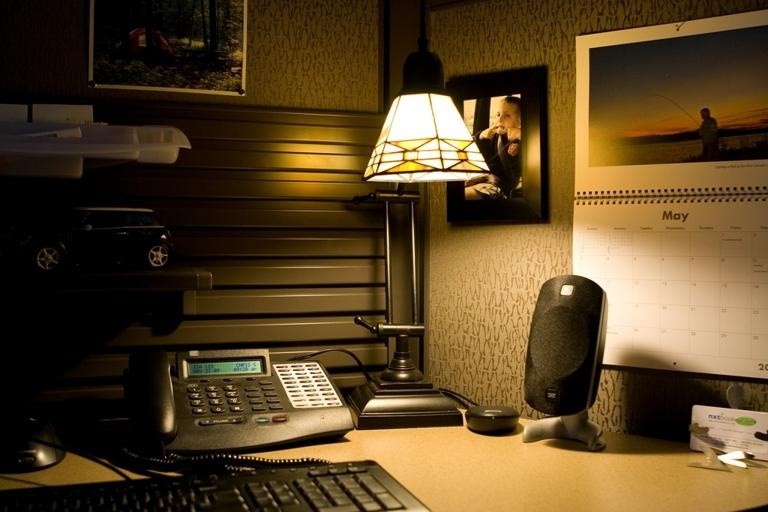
[522,274,608,452]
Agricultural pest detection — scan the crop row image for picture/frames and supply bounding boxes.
[88,2,249,95]
[446,65,548,223]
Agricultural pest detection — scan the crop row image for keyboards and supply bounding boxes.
[0,460,432,512]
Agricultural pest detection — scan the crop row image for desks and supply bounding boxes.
[0,407,766,512]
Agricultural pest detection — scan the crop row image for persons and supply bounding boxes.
[694,105,725,160]
[465,96,523,197]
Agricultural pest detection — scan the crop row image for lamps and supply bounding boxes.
[343,31,490,429]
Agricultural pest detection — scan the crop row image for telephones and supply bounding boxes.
[123,344,354,473]
